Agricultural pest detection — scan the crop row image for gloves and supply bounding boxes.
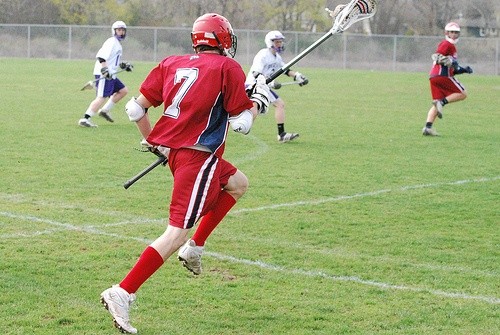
[101,67,113,80]
[293,72,309,87]
[142,141,171,164]
[249,74,274,114]
[446,56,458,68]
[267,79,282,89]
[454,64,473,75]
[119,62,134,72]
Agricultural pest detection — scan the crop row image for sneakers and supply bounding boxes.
[99,284,138,335]
[422,126,439,136]
[277,132,299,143]
[98,107,115,122]
[433,99,442,118]
[79,113,98,128]
[177,238,206,275]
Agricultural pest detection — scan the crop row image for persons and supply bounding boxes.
[422,23,473,136]
[100,13,270,334]
[79,21,133,127]
[245,30,309,143]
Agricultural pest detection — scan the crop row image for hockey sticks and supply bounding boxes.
[245,80,304,93]
[122,0,378,190]
[80,68,126,91]
[437,55,470,73]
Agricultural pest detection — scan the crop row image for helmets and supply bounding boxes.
[265,30,286,53]
[444,22,461,45]
[111,21,127,41]
[190,13,237,58]
